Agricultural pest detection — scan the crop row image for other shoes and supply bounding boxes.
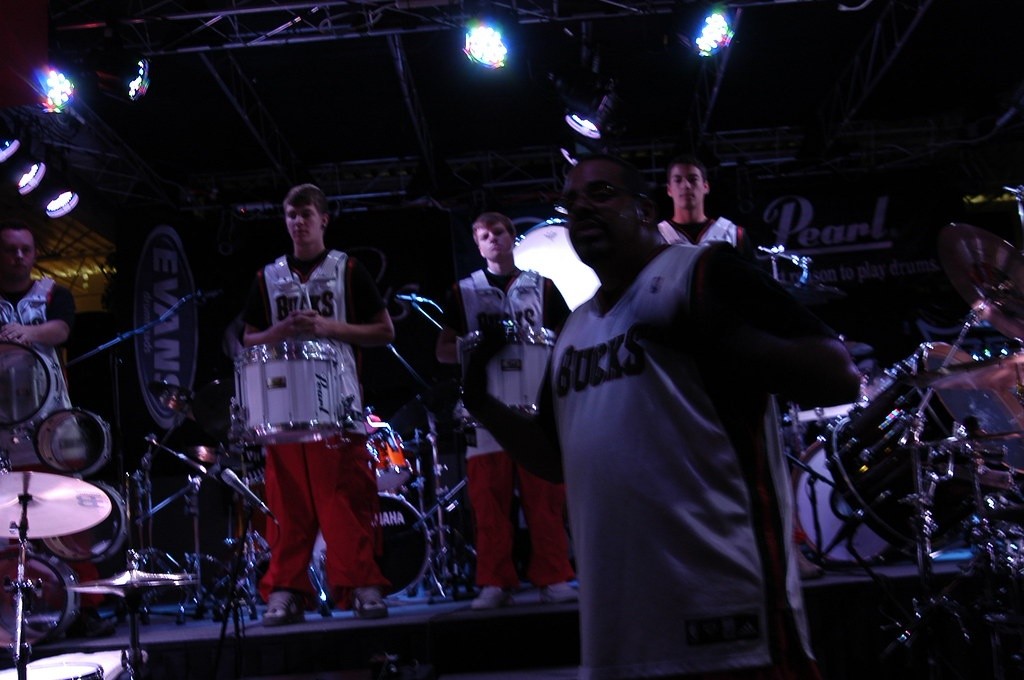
[538,582,578,605]
[261,590,306,627]
[352,586,389,621]
[471,585,514,609]
[66,608,114,638]
[796,547,823,583]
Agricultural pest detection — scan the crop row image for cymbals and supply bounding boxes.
[935,221,1023,347]
[63,570,201,595]
[188,445,259,467]
[786,401,853,422]
[150,380,226,435]
[0,469,114,540]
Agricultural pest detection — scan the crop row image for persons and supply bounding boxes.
[441,153,863,680]
[435,211,578,609]
[0,223,117,639]
[236,181,394,627]
[651,157,826,578]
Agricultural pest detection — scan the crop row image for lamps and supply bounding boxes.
[88,42,153,103]
[667,0,736,59]
[462,10,534,71]
[565,70,626,143]
[0,134,20,162]
[42,191,79,218]
[16,157,47,197]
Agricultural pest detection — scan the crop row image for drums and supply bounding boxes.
[790,433,899,570]
[193,378,232,441]
[1,546,79,651]
[454,328,553,428]
[0,339,62,435]
[231,338,349,445]
[33,408,113,475]
[2,662,103,680]
[823,341,993,556]
[358,419,414,492]
[42,481,134,564]
[374,493,433,600]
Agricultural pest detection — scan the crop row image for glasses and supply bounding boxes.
[553,184,647,215]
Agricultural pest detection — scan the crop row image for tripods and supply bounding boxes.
[410,401,479,605]
[127,437,261,628]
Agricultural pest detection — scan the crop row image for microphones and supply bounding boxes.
[397,294,433,304]
[181,289,206,302]
